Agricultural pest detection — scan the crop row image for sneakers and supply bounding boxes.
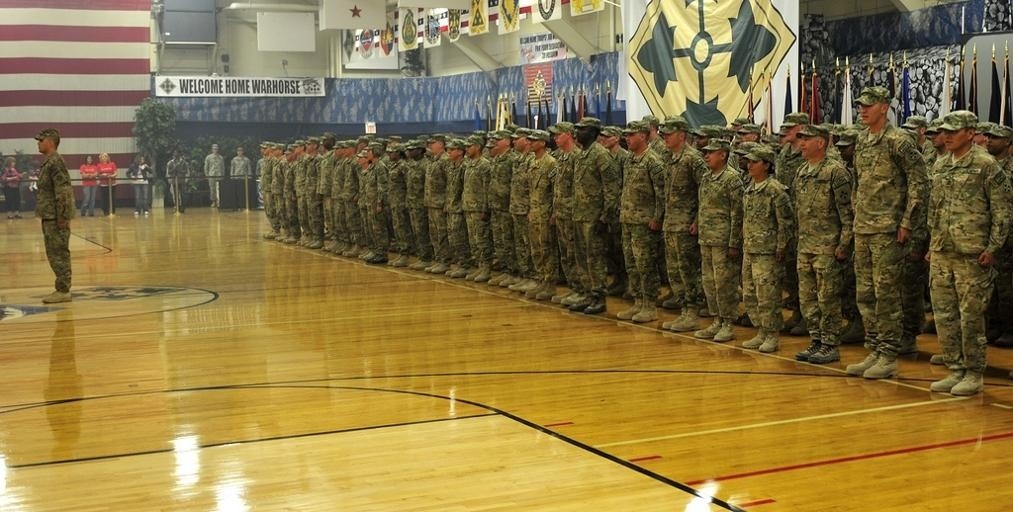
[262,228,391,264]
[931,353,945,364]
[737,312,917,378]
[550,290,607,314]
[931,372,985,395]
[391,254,557,301]
[43,292,72,303]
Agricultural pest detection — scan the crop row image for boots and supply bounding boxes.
[605,279,735,341]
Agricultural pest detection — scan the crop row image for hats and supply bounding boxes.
[261,111,1012,163]
[35,128,59,140]
[853,87,891,106]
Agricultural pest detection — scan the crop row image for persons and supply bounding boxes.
[230,147,252,178]
[165,151,189,212]
[96,153,119,218]
[257,112,1012,395]
[78,156,99,216]
[845,87,928,379]
[125,153,155,215]
[203,144,226,208]
[33,129,74,304]
[0,157,24,218]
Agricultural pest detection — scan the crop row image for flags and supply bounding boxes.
[475,92,616,135]
[747,54,1012,137]
